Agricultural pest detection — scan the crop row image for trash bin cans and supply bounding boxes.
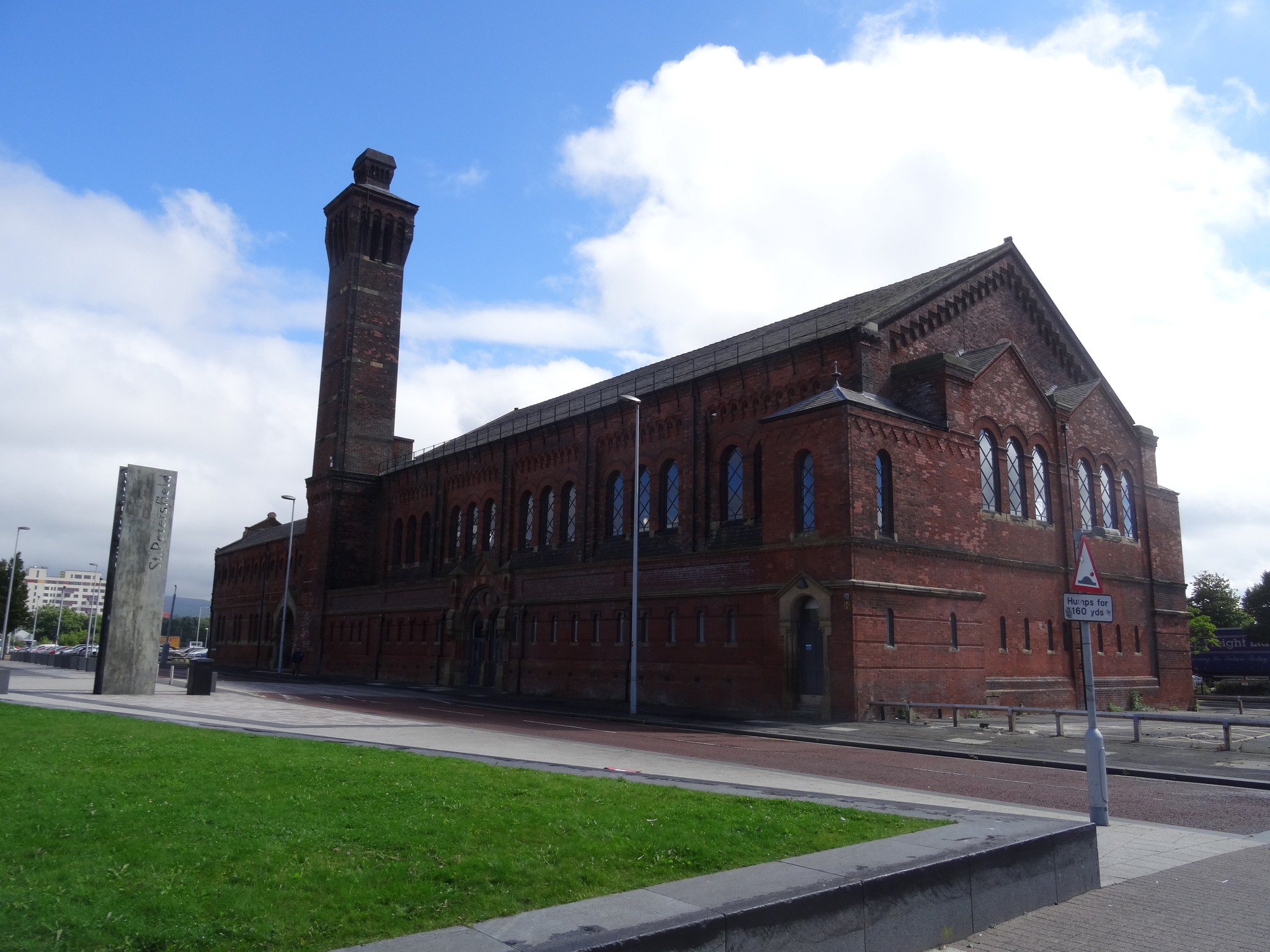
[187,657,215,695]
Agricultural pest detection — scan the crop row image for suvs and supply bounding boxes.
[0,644,99,657]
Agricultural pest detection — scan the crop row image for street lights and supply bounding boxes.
[91,577,103,657]
[278,495,296,673]
[84,563,98,658]
[160,585,177,668]
[54,586,67,654]
[618,394,642,715]
[30,595,41,653]
[0,526,30,660]
[204,628,209,648]
[196,606,209,647]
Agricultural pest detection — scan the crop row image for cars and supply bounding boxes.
[158,645,208,661]
